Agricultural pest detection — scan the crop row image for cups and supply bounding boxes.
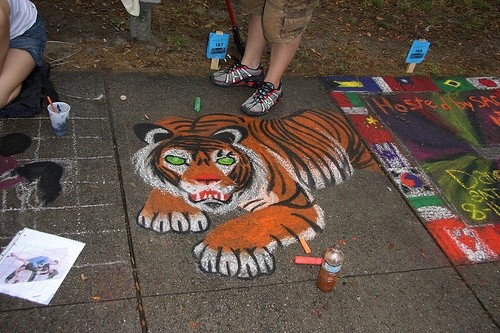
[48,101,70,136]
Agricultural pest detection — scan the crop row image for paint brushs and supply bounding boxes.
[47,96,57,113]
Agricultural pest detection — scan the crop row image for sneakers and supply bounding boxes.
[211,57,265,87]
[240,82,283,116]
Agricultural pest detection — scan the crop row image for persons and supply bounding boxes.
[210,0,315,116]
[0,0,59,111]
[5,251,59,286]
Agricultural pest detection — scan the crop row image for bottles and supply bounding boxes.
[317,243,345,292]
